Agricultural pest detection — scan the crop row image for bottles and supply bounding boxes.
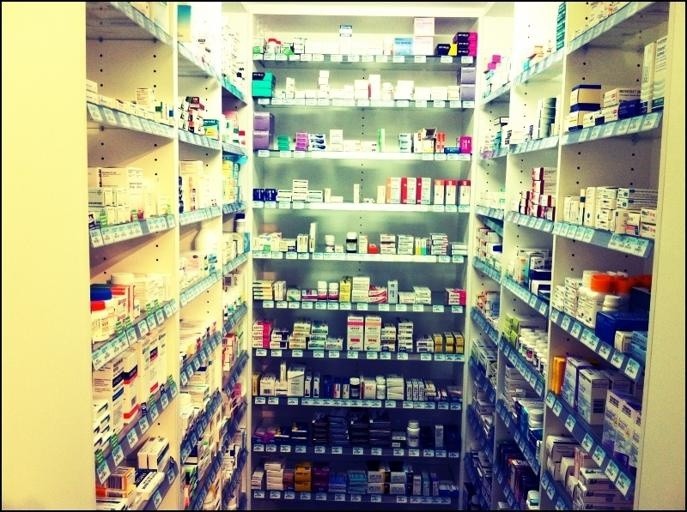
[518,328,532,354]
[253,188,264,200]
[323,376,331,398]
[578,196,586,225]
[90,300,110,342]
[112,291,124,331]
[329,283,338,300]
[575,270,598,323]
[527,409,543,442]
[526,335,540,361]
[519,474,535,510]
[469,440,486,467]
[535,344,547,370]
[539,348,547,375]
[535,329,545,335]
[90,283,116,336]
[343,377,350,399]
[350,377,360,399]
[436,132,445,153]
[445,147,460,153]
[602,294,621,314]
[264,189,275,201]
[529,500,539,511]
[513,468,530,502]
[346,233,357,253]
[522,332,535,357]
[613,275,632,313]
[276,41,281,55]
[266,38,277,55]
[325,235,335,253]
[539,332,548,339]
[406,420,420,448]
[317,280,327,301]
[526,489,538,510]
[113,287,127,326]
[377,378,386,400]
[587,274,614,328]
[469,495,480,511]
[110,273,134,323]
[460,136,470,153]
[542,336,548,343]
[332,377,340,398]
[423,128,436,153]
[532,339,545,366]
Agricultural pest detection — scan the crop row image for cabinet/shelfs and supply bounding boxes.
[463,9,685,503]
[2,0,252,508]
[252,8,483,508]
